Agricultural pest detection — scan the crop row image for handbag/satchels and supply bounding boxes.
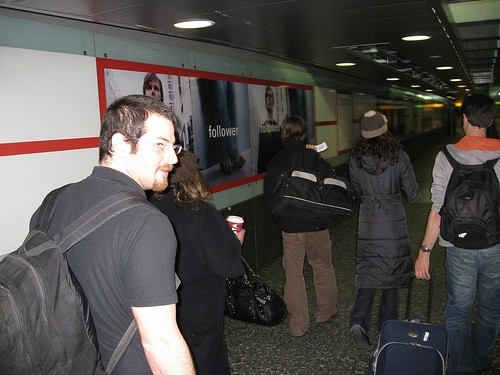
[272,147,354,220]
[224,255,289,326]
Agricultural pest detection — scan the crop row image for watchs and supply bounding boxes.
[419,243,432,252]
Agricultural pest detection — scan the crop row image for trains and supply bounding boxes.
[0,7,460,267]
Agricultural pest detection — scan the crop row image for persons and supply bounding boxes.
[256,87,283,175]
[263,115,339,336]
[145,150,246,375]
[350,111,419,350]
[143,72,164,102]
[414,91,500,375]
[29,95,196,375]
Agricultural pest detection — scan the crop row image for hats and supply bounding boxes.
[168,144,198,183]
[360,111,388,138]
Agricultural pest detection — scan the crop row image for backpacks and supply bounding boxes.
[0,184,162,375]
[439,146,500,250]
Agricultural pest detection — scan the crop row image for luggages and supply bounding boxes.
[364,272,449,375]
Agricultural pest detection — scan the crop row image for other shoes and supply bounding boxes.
[351,326,371,349]
[332,306,340,317]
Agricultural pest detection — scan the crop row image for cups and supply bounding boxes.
[226,216,244,233]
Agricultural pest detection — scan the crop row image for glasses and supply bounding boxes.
[125,134,182,156]
